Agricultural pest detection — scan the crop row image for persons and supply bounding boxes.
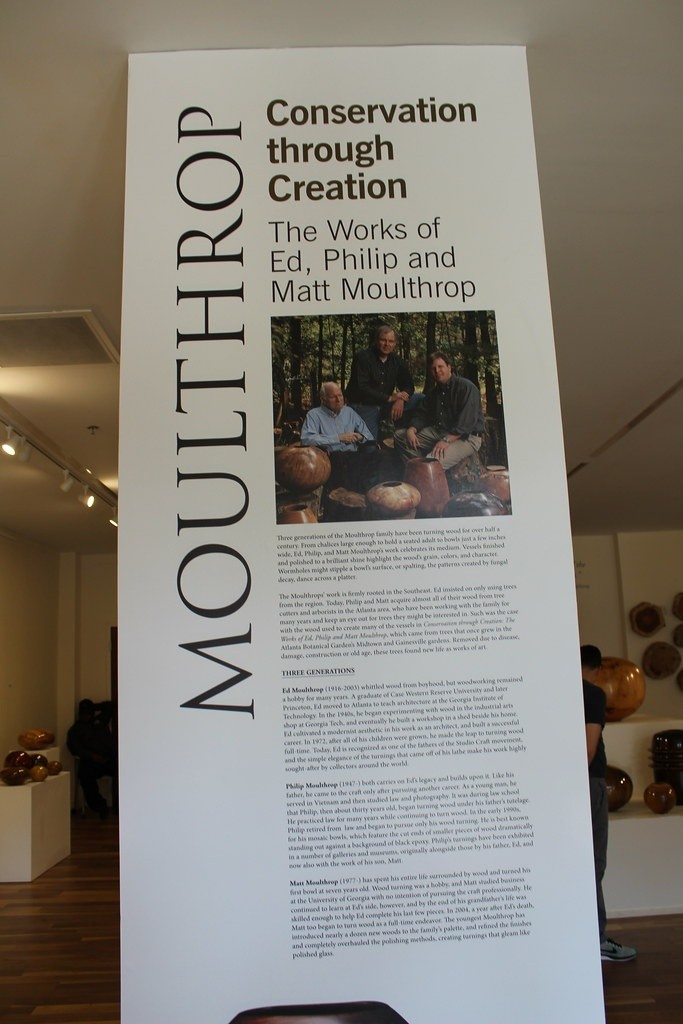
[345,325,415,440]
[395,352,483,473]
[581,643,637,963]
[67,699,118,814]
[299,381,375,514]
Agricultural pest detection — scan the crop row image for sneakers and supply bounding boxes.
[601,936,638,962]
